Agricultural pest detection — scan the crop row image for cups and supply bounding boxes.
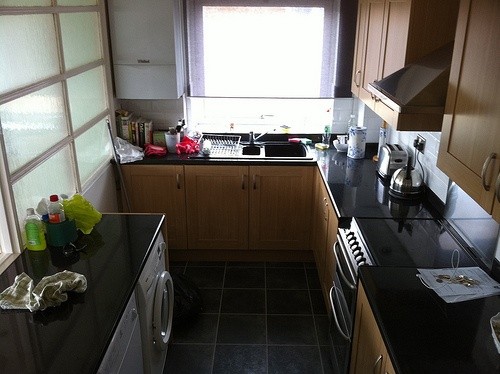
[165,132,180,154]
[40,212,78,246]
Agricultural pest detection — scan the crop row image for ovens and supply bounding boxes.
[325,216,374,374]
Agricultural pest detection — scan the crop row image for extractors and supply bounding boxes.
[363,42,456,117]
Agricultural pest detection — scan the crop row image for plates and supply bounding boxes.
[489,310,500,354]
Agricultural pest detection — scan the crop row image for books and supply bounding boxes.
[115,110,153,149]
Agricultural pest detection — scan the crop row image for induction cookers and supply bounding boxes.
[353,178,500,269]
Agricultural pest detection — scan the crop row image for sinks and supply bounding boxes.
[265,143,313,160]
[242,147,260,155]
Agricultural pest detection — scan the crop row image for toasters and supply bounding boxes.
[375,143,410,181]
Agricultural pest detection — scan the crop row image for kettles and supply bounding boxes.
[390,156,424,196]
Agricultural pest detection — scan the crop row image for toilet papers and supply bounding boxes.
[346,126,367,160]
[377,127,388,158]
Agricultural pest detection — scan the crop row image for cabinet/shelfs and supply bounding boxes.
[321,197,340,317]
[351,0,412,131]
[436,0,500,227]
[313,166,328,307]
[113,165,188,263]
[185,165,313,263]
[348,279,395,374]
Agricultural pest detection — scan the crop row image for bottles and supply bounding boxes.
[22,208,46,252]
[321,116,389,161]
[47,195,65,222]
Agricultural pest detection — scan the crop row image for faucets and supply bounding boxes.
[240,130,268,145]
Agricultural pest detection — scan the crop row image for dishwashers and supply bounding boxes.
[96,291,145,374]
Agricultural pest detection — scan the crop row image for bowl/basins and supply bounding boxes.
[333,138,350,153]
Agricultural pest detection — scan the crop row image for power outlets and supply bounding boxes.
[413,134,426,153]
